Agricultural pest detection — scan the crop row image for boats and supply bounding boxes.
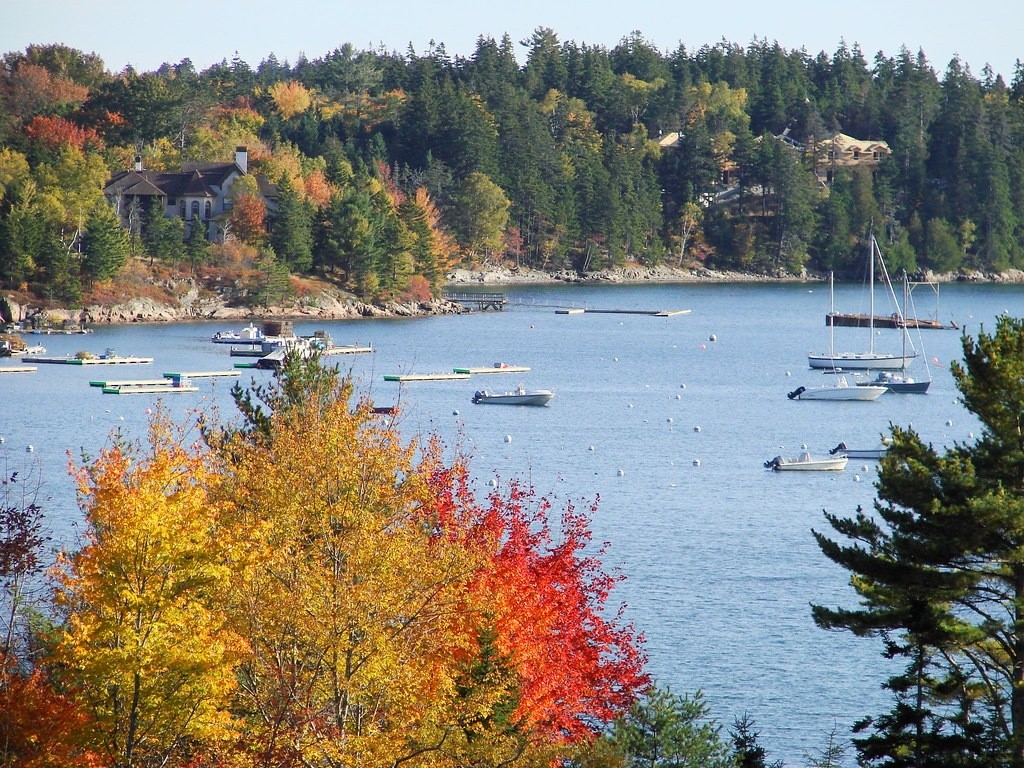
[787,219,932,401]
[825,278,942,329]
[763,454,850,470]
[454,362,531,374]
[163,370,242,377]
[0,367,37,371]
[90,378,198,394]
[830,442,888,458]
[383,374,471,381]
[472,380,554,406]
[22,356,155,364]
[357,404,394,413]
[212,320,372,369]
